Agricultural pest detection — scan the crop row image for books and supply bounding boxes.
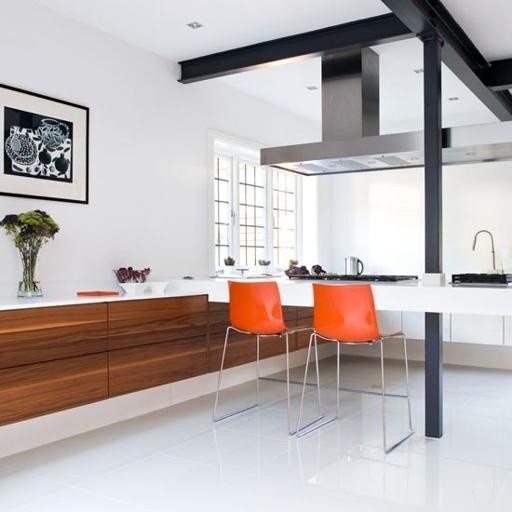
[76,291,119,298]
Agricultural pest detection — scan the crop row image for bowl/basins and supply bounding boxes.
[148,282,169,295]
[118,282,149,295]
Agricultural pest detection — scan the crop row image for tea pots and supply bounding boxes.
[344,256,364,276]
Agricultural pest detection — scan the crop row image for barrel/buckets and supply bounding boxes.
[473,229,496,273]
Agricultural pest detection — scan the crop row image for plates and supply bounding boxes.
[5,134,37,165]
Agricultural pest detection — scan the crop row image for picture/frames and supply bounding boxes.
[1,82,91,205]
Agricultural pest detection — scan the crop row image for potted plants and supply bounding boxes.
[0,208,60,299]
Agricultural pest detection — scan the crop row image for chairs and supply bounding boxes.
[213,278,418,457]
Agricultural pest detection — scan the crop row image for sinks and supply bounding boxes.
[452,272,512,282]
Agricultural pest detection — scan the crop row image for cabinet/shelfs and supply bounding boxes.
[1,295,336,458]
